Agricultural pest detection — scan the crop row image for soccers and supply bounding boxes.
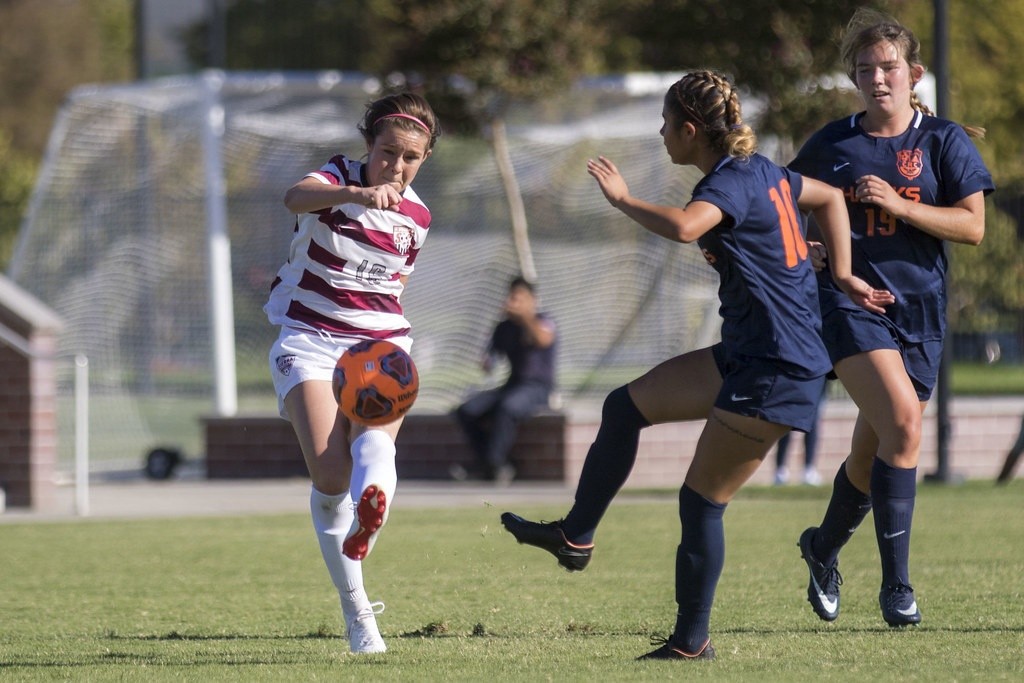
[332,339,419,424]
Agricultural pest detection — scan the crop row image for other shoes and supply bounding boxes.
[449,463,490,481]
[491,463,517,492]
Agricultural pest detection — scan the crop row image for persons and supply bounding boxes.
[447,274,559,483]
[263,93,442,654]
[501,68,897,659]
[774,383,827,486]
[786,10,997,626]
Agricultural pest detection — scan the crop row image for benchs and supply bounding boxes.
[197,411,569,482]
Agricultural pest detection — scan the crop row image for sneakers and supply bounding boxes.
[342,485,388,561]
[501,512,594,574]
[879,576,922,628]
[636,635,715,664]
[341,602,388,656]
[797,526,843,622]
